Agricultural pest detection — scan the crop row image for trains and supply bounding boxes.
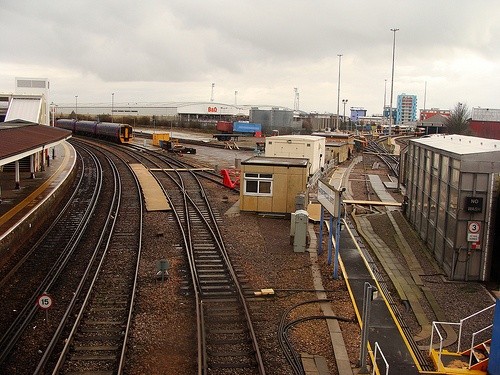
[56,119,133,144]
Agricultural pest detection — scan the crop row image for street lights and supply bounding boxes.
[75,95,78,120]
[336,54,343,132]
[342,99,348,133]
[390,28,399,136]
